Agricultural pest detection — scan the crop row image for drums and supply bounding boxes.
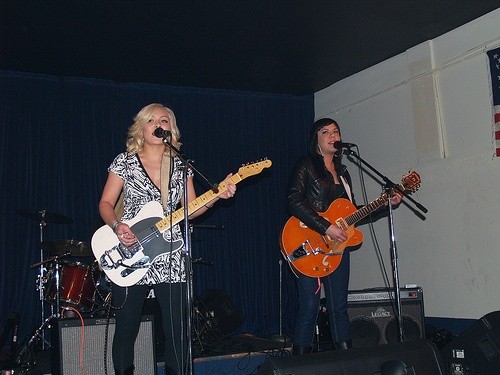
[100,292,112,308]
[41,259,100,312]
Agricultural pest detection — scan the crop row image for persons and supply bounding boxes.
[288,118,404,355]
[99,103,237,375]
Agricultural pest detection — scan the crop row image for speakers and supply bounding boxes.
[255,286,500,375]
[50,313,156,375]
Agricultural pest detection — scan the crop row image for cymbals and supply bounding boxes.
[34,239,93,257]
[15,208,74,225]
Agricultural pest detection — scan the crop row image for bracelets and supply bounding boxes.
[111,221,120,233]
[204,204,213,208]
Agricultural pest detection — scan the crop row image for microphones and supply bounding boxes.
[333,140,356,149]
[154,128,171,138]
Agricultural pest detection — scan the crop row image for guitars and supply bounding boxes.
[279,171,421,279]
[91,157,272,287]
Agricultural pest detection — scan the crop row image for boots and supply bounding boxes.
[333,338,353,351]
[292,343,313,356]
